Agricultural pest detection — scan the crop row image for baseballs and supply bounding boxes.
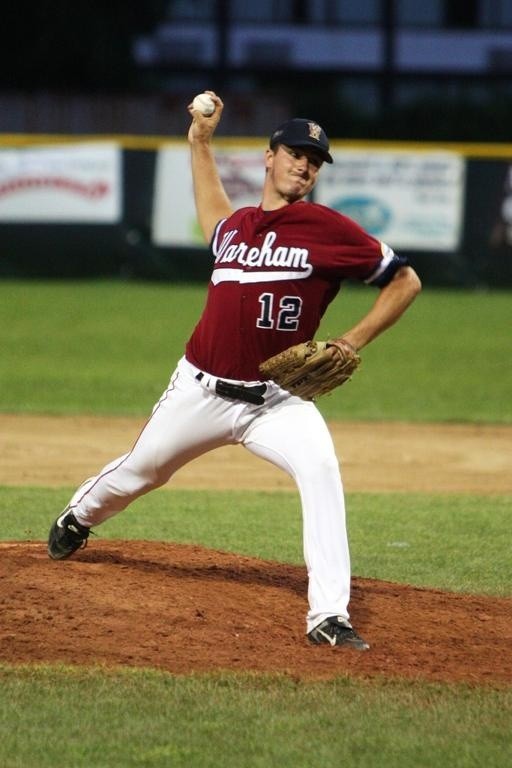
[192,93,215,118]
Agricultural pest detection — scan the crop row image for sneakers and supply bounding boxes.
[305,616,370,650]
[48,476,97,560]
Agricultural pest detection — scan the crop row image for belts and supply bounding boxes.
[195,372,267,405]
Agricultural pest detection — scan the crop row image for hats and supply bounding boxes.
[269,117,333,164]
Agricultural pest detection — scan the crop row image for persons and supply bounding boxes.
[42,88,424,654]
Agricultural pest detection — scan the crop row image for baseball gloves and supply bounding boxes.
[259,339,360,400]
